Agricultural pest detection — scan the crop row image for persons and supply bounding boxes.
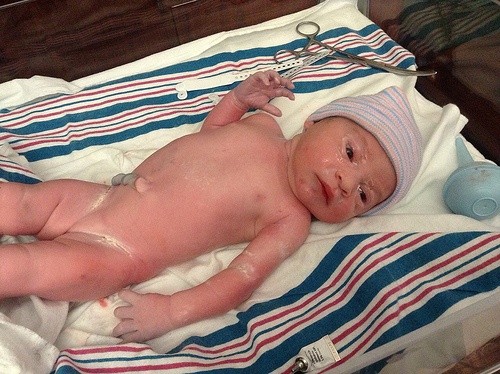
[0,69,424,344]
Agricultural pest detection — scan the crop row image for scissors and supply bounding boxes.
[274,21,437,76]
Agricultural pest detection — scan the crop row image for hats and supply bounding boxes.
[300,84,426,218]
